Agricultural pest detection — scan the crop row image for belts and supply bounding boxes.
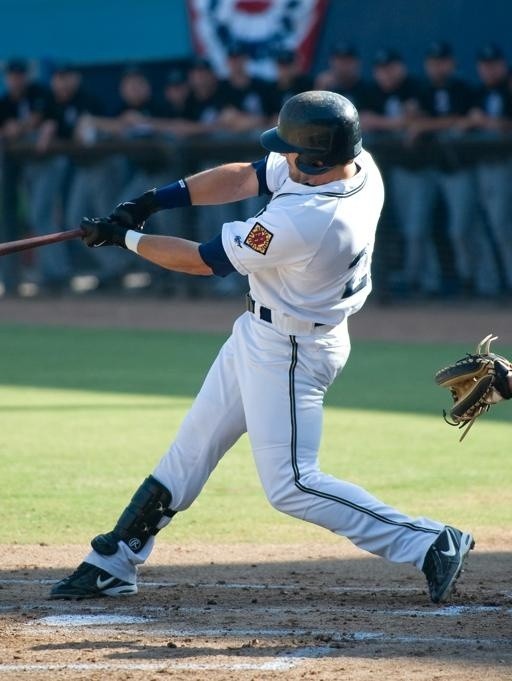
[243,289,326,329]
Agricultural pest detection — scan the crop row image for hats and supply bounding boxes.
[9,57,28,74]
[49,61,83,76]
[121,46,506,77]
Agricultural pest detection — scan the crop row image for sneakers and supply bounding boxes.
[422,525,475,605]
[44,563,138,599]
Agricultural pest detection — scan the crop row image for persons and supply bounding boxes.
[47,91,477,605]
[1,44,512,300]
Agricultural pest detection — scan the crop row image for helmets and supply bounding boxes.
[260,90,364,176]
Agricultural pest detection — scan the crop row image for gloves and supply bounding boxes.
[77,196,147,250]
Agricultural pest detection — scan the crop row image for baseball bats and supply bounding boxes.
[0,229,88,255]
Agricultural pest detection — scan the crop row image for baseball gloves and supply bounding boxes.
[435,352,512,424]
